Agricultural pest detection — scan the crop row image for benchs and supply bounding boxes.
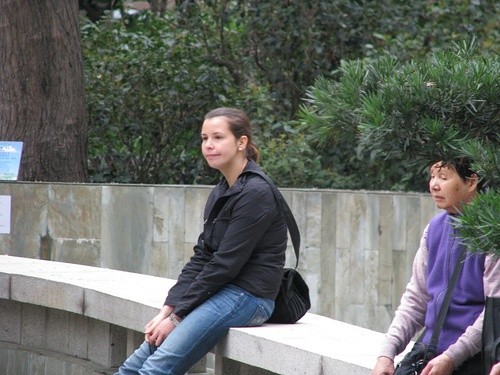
[0,253,413,375]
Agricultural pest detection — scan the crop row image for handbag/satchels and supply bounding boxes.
[264,267,311,325]
[393,345,439,375]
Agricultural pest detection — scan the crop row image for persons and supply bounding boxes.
[374,147,500,375]
[114,107,287,375]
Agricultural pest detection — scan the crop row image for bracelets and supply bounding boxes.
[169,314,180,326]
